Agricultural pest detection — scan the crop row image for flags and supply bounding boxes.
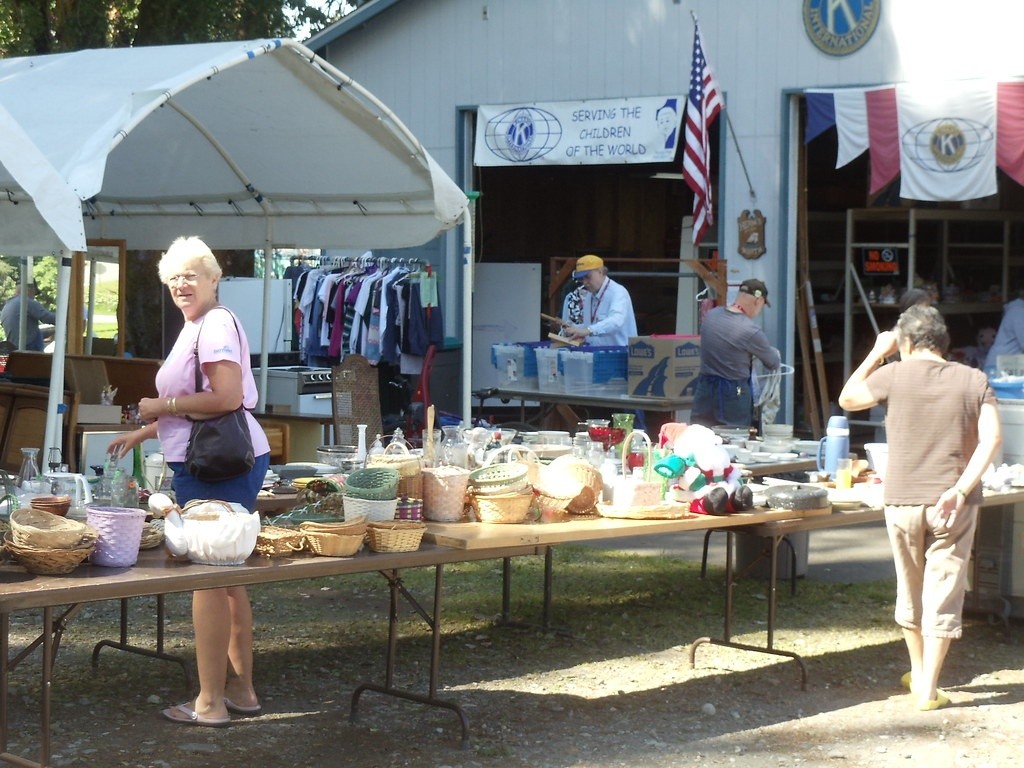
[682,22,725,249]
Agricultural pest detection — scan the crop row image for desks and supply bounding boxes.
[0,453,1024,768]
[492,386,692,444]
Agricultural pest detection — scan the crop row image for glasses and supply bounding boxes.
[165,272,206,284]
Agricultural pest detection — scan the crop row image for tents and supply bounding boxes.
[0,38,475,475]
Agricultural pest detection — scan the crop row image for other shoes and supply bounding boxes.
[919,689,951,711]
[901,671,922,694]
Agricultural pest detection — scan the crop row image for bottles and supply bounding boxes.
[387,427,406,454]
[15,448,41,494]
[440,425,461,465]
[112,471,125,507]
[367,434,384,462]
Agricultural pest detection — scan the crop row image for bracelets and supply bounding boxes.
[954,484,966,497]
[166,395,178,416]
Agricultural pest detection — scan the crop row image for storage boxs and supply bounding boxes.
[490,335,701,399]
[77,405,122,424]
[265,403,292,416]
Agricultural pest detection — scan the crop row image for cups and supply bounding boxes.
[103,452,119,479]
[612,413,636,460]
[586,420,610,427]
[422,429,441,460]
[495,428,516,447]
[837,458,852,491]
[736,450,750,463]
[588,428,626,449]
[731,439,760,452]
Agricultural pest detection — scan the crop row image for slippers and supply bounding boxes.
[162,702,231,727]
[222,697,262,715]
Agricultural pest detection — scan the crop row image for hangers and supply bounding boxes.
[696,272,712,301]
[297,254,422,291]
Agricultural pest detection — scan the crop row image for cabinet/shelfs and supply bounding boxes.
[794,208,1024,448]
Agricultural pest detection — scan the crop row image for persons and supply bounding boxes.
[0,274,55,352]
[838,289,1003,711]
[983,284,1024,379]
[556,255,650,441]
[690,278,782,427]
[105,236,271,727]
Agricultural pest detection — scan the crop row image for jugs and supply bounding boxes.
[43,472,93,520]
[816,416,850,482]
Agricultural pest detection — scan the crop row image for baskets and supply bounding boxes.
[1,494,162,572]
[253,435,602,558]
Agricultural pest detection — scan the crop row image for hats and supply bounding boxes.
[573,255,603,279]
[16,273,42,296]
[739,278,771,308]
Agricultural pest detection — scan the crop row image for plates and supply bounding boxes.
[774,453,797,460]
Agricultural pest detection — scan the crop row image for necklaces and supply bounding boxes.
[732,303,746,314]
[591,279,610,326]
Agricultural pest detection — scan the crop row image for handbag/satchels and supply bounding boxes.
[185,401,255,483]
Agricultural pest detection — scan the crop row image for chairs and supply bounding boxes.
[330,355,386,452]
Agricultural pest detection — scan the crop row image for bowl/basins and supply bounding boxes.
[763,424,793,438]
[752,452,771,461]
[30,497,71,517]
[711,424,753,438]
[794,440,826,455]
[722,444,738,462]
